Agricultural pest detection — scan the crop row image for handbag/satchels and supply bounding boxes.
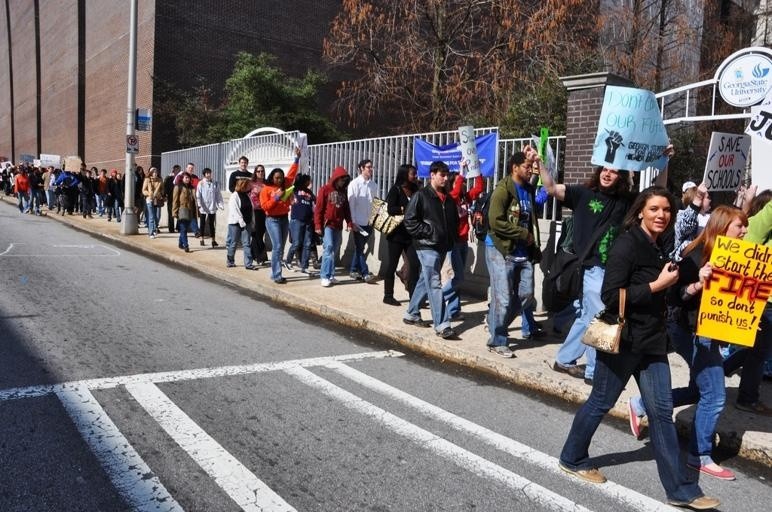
[154,196,165,207]
[368,197,404,235]
[542,250,584,311]
[581,316,626,355]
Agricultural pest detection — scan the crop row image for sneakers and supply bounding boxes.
[383,295,469,338]
[553,361,585,379]
[558,461,606,483]
[735,396,771,416]
[625,398,643,440]
[686,459,737,480]
[486,344,514,358]
[178,240,219,252]
[667,496,721,509]
[226,259,378,287]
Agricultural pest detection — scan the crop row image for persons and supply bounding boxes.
[1,163,124,222]
[133,163,225,252]
[227,148,550,357]
[522,145,772,511]
